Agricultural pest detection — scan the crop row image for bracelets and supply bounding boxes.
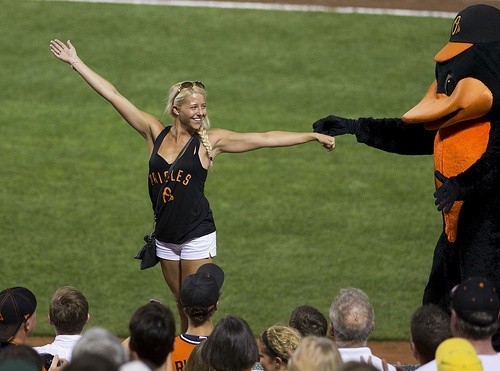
[70,61,83,70]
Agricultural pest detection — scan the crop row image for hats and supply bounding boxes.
[178,263,225,315]
[435,337,483,371]
[448,277,500,327]
[0,286,37,343]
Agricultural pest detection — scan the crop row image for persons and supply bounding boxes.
[0,263,500,371]
[50,38,336,335]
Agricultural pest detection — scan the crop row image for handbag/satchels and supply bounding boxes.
[133,236,160,270]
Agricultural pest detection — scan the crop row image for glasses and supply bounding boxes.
[172,82,205,106]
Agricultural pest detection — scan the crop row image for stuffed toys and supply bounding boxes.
[313,5,500,314]
